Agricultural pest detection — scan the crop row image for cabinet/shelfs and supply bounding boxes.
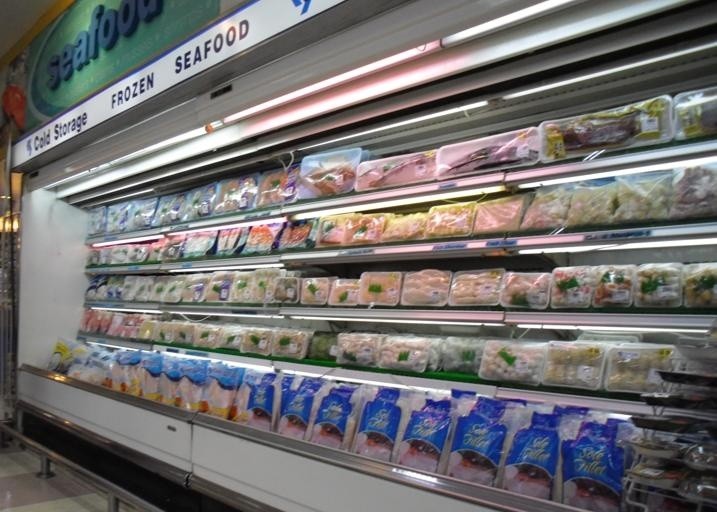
[11,0,717,512]
